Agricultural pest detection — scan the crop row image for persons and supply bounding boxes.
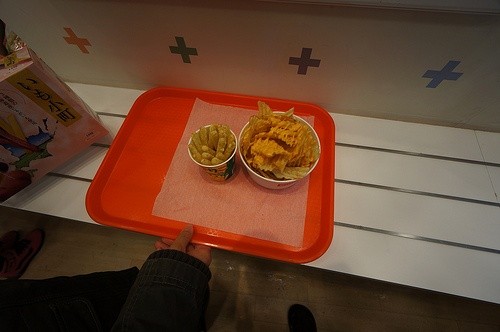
[0,224,320,332]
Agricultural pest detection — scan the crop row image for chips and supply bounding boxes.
[241,101,320,181]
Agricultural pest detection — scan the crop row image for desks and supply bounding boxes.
[0,83,500,309]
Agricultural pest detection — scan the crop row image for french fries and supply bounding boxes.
[189,124,236,165]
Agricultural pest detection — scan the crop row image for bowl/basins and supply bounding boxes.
[237,111,321,190]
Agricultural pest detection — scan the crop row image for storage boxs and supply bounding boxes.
[0,47,111,206]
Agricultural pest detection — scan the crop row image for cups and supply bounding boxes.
[188,124,237,183]
